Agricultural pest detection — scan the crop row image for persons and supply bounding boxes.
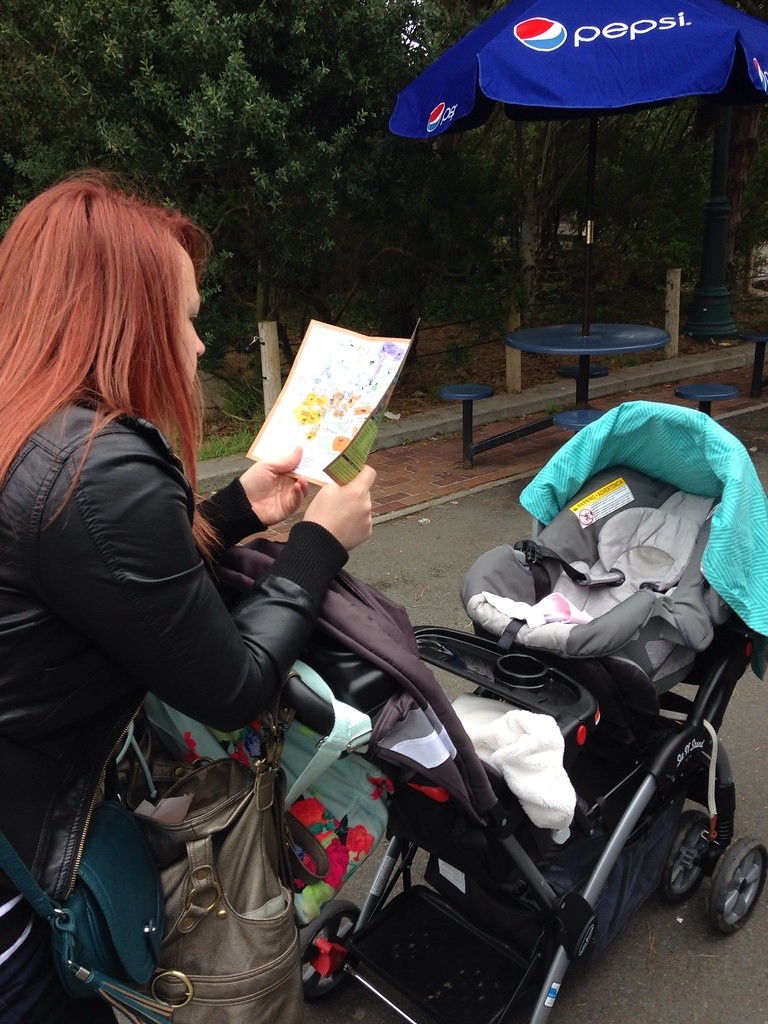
[0,180,375,1024]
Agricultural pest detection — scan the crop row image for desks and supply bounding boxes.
[474,322,675,466]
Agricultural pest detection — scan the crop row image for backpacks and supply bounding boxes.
[143,656,390,927]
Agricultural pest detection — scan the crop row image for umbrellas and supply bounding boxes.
[389,0,768,337]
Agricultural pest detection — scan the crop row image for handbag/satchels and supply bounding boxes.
[112,726,305,1024]
[57,805,175,1024]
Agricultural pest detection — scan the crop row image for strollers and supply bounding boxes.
[221,398,768,1024]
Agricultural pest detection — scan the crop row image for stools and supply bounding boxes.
[739,333,768,399]
[674,383,737,418]
[441,385,495,470]
[552,409,605,436]
[555,365,609,381]
